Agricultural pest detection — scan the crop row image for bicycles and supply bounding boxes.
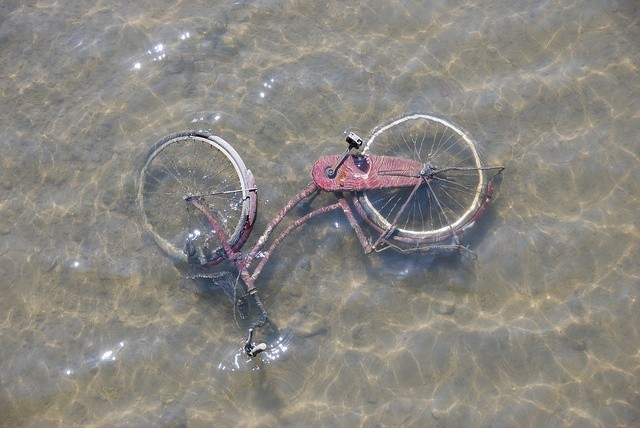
[134,111,505,357]
